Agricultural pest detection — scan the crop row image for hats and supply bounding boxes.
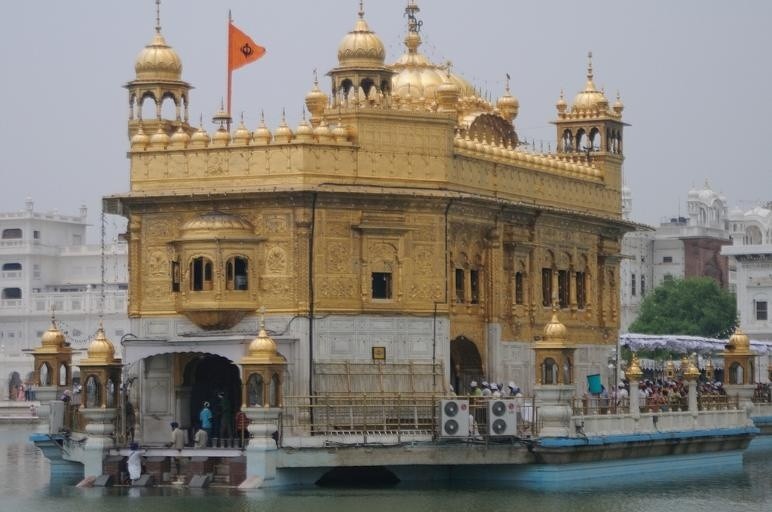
[470,381,503,391]
[509,382,518,393]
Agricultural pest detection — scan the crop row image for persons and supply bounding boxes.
[613,375,725,412]
[448,378,526,425]
[198,390,249,448]
[190,422,208,448]
[164,420,187,474]
[10,381,36,402]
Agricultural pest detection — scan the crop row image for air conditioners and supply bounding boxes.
[436,399,519,440]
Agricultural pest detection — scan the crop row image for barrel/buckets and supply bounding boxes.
[587,373,603,394]
